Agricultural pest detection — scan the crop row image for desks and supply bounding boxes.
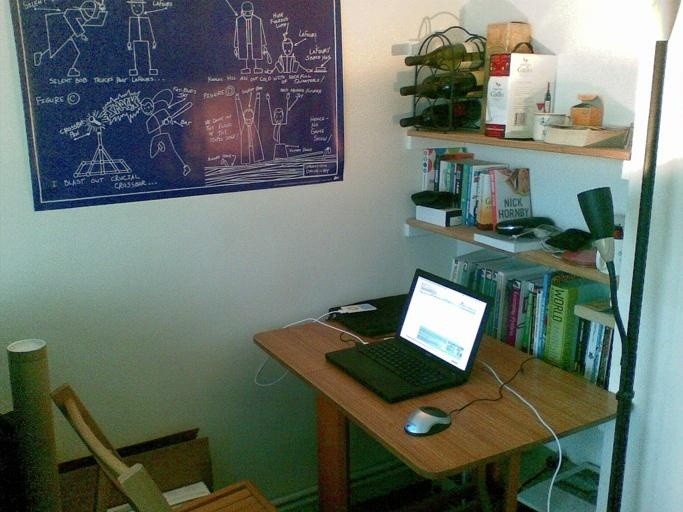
[253,318,635,511]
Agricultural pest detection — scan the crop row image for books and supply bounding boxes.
[544,124,629,147]
[415,147,548,254]
[516,462,600,512]
[449,249,615,392]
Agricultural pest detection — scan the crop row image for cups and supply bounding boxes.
[485,52,510,138]
[536,103,544,113]
[596,236,622,276]
[534,112,571,140]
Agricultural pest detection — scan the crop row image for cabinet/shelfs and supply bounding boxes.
[406,128,630,289]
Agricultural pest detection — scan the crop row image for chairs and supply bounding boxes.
[50,383,278,512]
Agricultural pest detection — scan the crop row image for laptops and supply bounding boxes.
[324,266,494,403]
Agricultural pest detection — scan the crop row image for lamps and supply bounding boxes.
[576,40,682,511]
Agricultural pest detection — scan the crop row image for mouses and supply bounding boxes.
[404,406,451,436]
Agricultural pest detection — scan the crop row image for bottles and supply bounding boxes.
[544,82,551,113]
[398,41,484,127]
